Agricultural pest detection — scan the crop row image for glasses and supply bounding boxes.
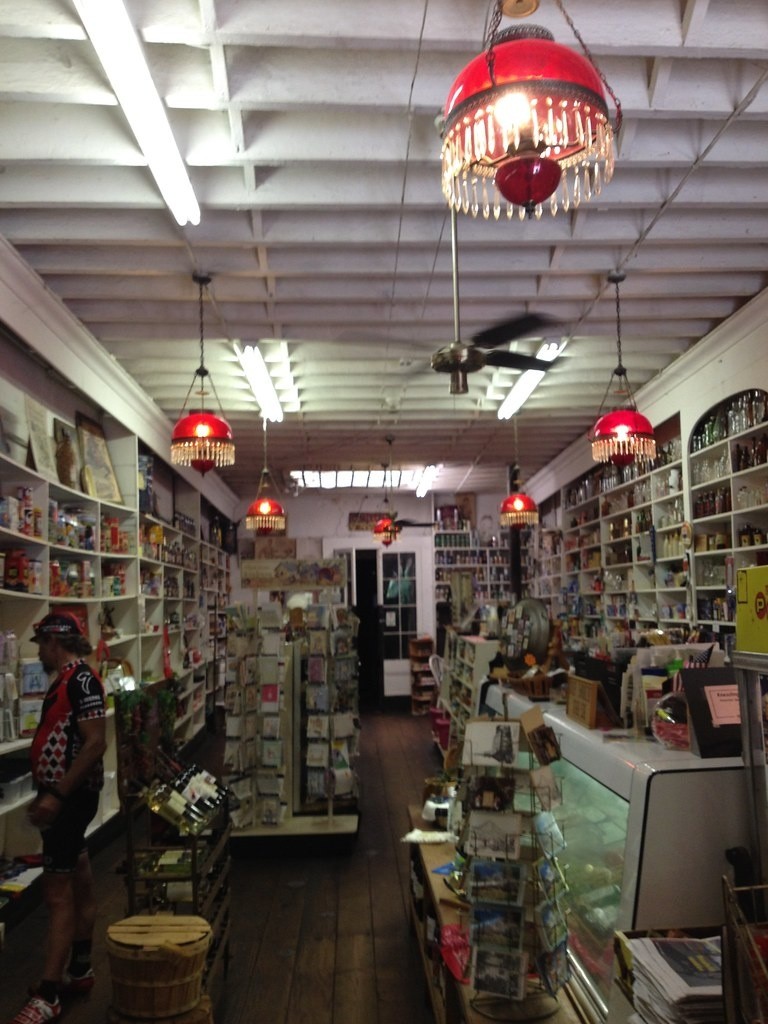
[32,618,67,633]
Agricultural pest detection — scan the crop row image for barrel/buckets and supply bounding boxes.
[104,909,213,1019]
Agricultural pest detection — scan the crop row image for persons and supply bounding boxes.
[9,610,111,1024]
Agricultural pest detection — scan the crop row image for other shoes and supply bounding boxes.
[28,965,96,996]
[8,993,62,1024]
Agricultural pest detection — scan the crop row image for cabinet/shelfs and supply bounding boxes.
[226,583,360,838]
[519,285,768,643]
[0,229,207,942]
[107,677,234,992]
[432,529,528,655]
[409,639,434,715]
[198,469,240,714]
[406,803,591,1024]
[429,632,501,757]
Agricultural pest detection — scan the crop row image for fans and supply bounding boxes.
[385,434,437,528]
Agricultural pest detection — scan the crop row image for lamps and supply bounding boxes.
[170,272,237,479]
[496,335,562,420]
[416,463,436,498]
[73,0,202,228]
[498,407,539,527]
[240,339,284,422]
[588,269,657,468]
[373,463,402,547]
[430,0,624,217]
[246,416,287,531]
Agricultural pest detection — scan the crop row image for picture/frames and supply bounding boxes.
[75,411,126,505]
[454,492,477,528]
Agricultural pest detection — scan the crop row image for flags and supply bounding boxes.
[672,645,715,693]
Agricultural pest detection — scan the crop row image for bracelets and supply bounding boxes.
[48,787,66,802]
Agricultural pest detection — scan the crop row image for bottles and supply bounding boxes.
[636,506,651,534]
[436,533,470,547]
[652,469,682,500]
[735,433,768,472]
[565,470,601,509]
[600,461,618,493]
[435,585,449,600]
[434,520,443,531]
[693,486,732,519]
[458,519,471,530]
[474,567,487,582]
[692,450,727,486]
[490,566,510,582]
[623,463,640,482]
[658,496,683,528]
[648,441,673,470]
[624,540,631,563]
[691,389,768,453]
[435,568,455,581]
[662,530,684,557]
[490,584,510,599]
[130,754,228,835]
[739,523,763,547]
[436,550,487,564]
[473,584,487,599]
[712,597,728,621]
[490,550,508,564]
[623,517,630,537]
[601,475,650,517]
[608,522,614,541]
[737,482,768,510]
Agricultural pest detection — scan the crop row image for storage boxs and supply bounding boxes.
[29,559,43,595]
[4,549,29,593]
[436,719,450,750]
[429,705,450,731]
[17,697,44,737]
[76,559,92,598]
[20,657,48,693]
[0,496,19,531]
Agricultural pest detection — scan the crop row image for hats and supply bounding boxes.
[29,612,87,643]
